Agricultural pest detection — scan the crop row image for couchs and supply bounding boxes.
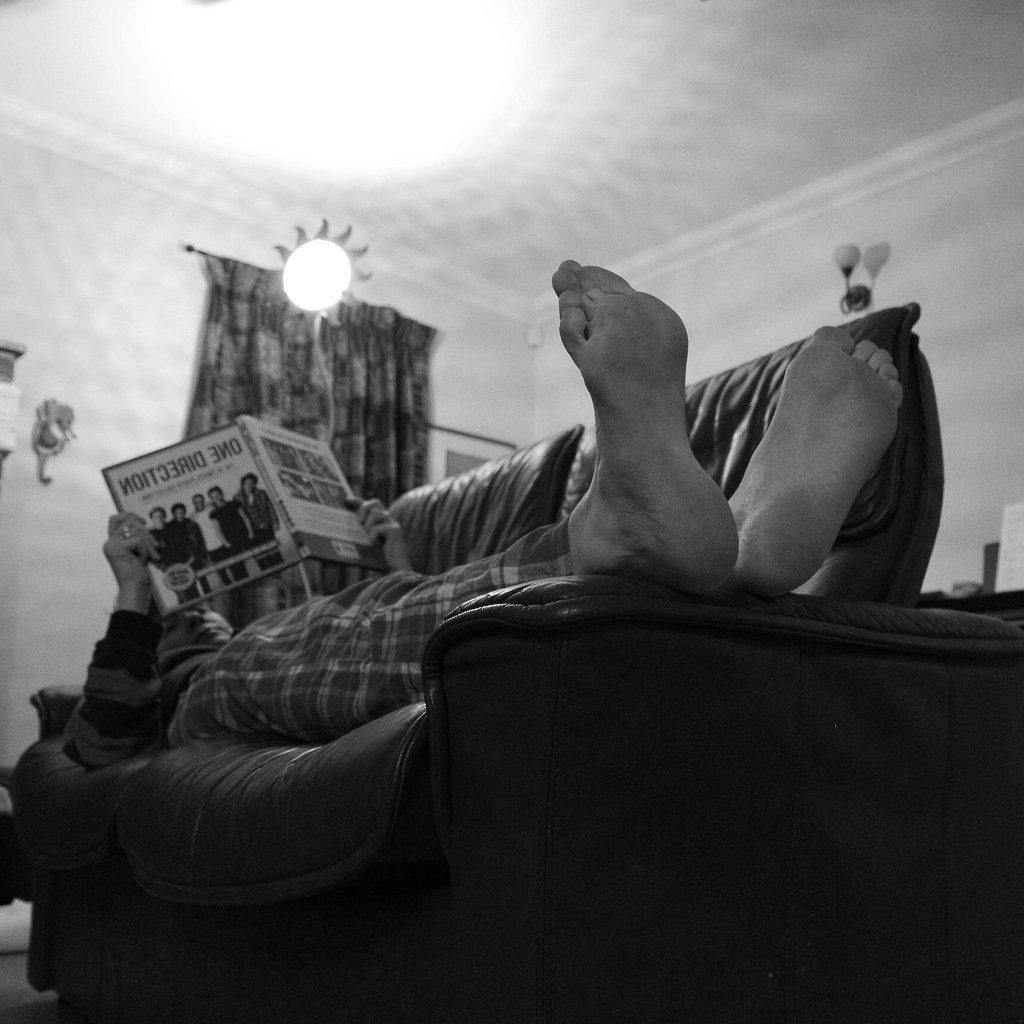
[8,301,1024,1023]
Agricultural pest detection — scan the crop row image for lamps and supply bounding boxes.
[835,243,871,316]
[276,216,374,439]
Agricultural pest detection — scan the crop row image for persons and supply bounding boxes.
[144,473,286,604]
[62,259,903,768]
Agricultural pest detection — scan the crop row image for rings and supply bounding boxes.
[120,527,130,540]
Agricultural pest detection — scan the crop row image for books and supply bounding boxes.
[102,413,388,618]
